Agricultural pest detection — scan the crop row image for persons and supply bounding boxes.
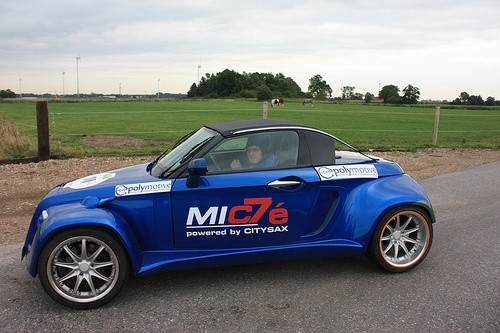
[230,134,275,169]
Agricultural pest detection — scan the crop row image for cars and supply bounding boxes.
[21,118,438,310]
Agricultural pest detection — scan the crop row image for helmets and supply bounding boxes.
[245,134,272,159]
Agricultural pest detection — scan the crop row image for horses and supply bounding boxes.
[271,98,285,108]
[302,99,314,107]
